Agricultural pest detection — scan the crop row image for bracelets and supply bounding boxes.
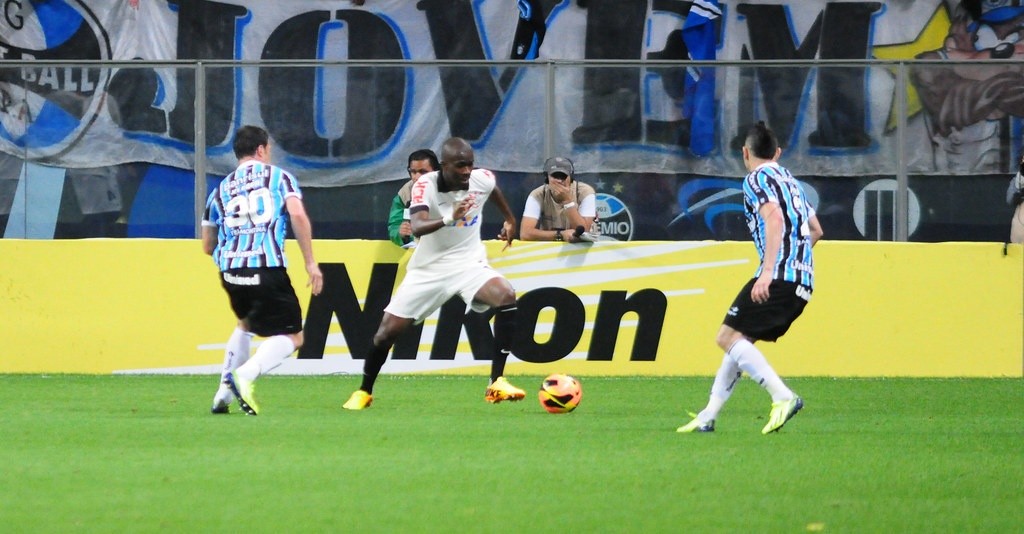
[565,202,575,209]
[442,213,455,224]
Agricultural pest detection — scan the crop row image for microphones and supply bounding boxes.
[573,226,585,237]
[401,200,411,244]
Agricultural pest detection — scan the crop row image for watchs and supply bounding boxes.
[553,229,563,241]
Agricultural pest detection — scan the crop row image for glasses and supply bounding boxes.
[406,166,429,175]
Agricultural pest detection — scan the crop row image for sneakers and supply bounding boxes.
[224,371,259,415]
[676,409,716,433]
[342,390,373,410]
[211,401,228,414]
[485,376,525,403]
[761,393,803,436]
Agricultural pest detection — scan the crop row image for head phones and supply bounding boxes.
[544,158,576,183]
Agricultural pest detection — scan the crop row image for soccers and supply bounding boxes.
[539,374,582,413]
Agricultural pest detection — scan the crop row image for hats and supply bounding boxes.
[544,157,572,175]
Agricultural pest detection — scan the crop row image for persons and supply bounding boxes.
[343,138,526,410]
[675,127,823,435]
[387,150,598,248]
[202,125,323,415]
